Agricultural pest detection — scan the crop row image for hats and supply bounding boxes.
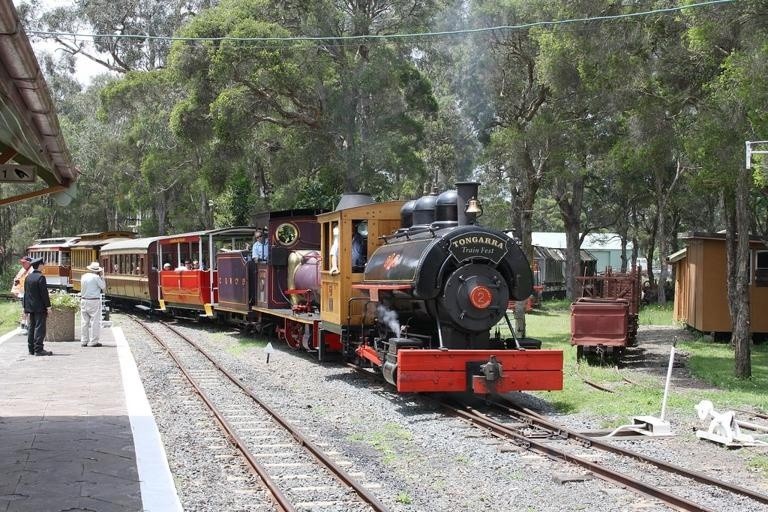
[86,262,103,272]
[20,257,32,263]
[29,257,47,266]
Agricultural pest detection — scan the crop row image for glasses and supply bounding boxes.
[257,233,262,239]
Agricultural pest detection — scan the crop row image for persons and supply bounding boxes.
[253,231,270,263]
[23,258,52,356]
[113,261,207,274]
[11,258,34,335]
[330,225,363,275]
[81,262,106,347]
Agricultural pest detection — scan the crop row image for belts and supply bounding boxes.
[81,297,100,300]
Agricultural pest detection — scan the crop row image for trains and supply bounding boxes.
[25,182,563,405]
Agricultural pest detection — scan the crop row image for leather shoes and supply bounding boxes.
[34,350,52,356]
[29,351,34,355]
[86,343,102,347]
